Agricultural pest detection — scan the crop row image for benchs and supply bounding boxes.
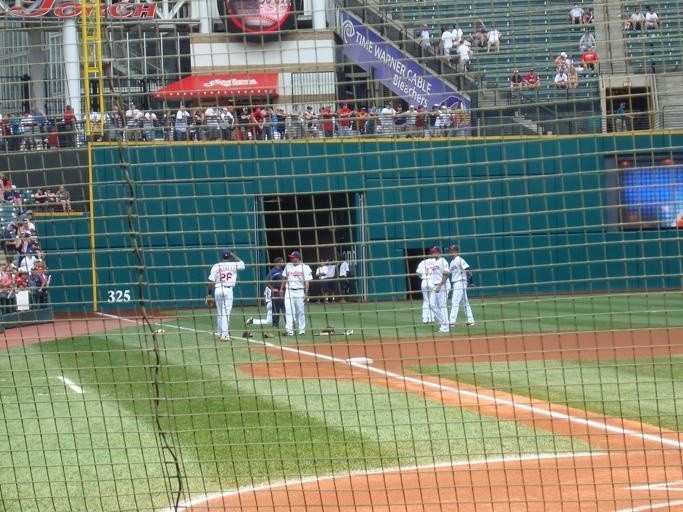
[377,0,683,100]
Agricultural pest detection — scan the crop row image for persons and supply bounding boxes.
[0,100,469,153]
[278,251,315,337]
[415,249,437,324]
[244,283,273,326]
[206,248,245,340]
[264,256,295,327]
[416,2,661,97]
[615,102,635,132]
[314,259,328,304]
[338,256,350,303]
[0,170,73,312]
[429,245,452,332]
[323,258,340,304]
[447,244,475,327]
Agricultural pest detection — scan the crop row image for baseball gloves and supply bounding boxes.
[206,296,214,306]
[243,332,253,337]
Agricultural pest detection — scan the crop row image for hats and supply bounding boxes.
[220,250,231,257]
[429,244,459,259]
[288,250,300,259]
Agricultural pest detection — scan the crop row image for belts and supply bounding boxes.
[223,286,231,288]
[288,288,303,290]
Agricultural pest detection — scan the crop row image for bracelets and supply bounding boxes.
[468,276,474,285]
[304,288,309,294]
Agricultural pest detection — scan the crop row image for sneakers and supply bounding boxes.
[246,317,253,325]
[217,335,231,341]
[273,324,306,336]
[422,320,476,334]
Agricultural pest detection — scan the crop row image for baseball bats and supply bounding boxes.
[313,333,344,336]
